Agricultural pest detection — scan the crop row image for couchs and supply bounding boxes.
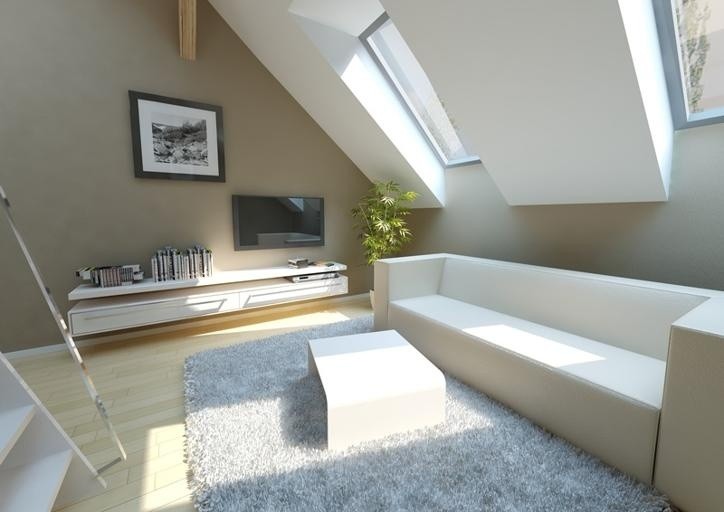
[373,250,723,512]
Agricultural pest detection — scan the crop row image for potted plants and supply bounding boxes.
[349,176,421,309]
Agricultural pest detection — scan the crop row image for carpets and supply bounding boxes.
[182,311,685,511]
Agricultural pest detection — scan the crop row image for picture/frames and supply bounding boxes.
[128,89,230,183]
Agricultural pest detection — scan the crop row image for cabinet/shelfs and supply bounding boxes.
[65,260,349,341]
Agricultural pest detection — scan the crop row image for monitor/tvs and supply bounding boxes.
[231,194,325,250]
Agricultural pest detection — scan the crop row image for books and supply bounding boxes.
[151,246,213,283]
